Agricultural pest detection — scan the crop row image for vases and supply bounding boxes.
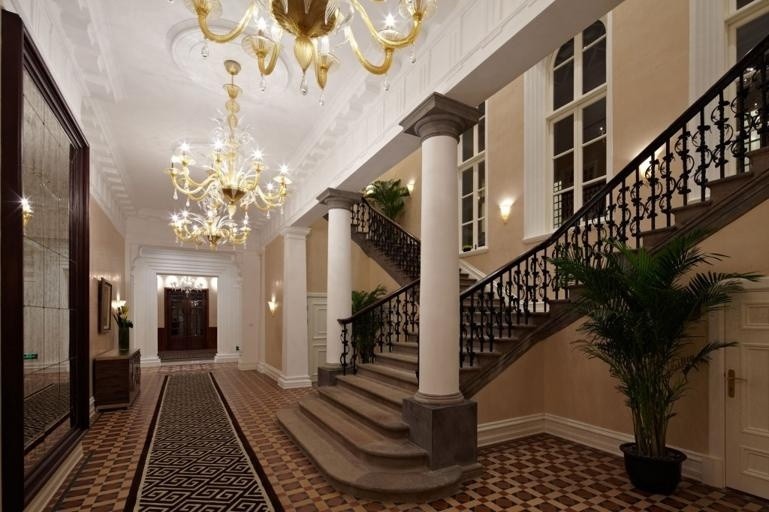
[119,326,129,351]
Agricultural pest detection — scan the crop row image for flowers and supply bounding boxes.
[113,304,134,329]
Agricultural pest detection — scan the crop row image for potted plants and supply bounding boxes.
[351,284,387,362]
[546,227,766,496]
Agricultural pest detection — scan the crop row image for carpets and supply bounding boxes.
[24,382,72,457]
[123,372,285,512]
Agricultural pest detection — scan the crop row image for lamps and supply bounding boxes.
[639,163,653,185]
[165,59,293,220]
[501,206,512,223]
[267,301,277,317]
[170,276,202,296]
[183,1,436,107]
[114,300,126,316]
[406,183,414,198]
[22,196,32,231]
[169,147,252,252]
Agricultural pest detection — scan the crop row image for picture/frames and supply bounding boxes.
[97,277,112,335]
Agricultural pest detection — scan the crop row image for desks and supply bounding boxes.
[94,347,141,410]
